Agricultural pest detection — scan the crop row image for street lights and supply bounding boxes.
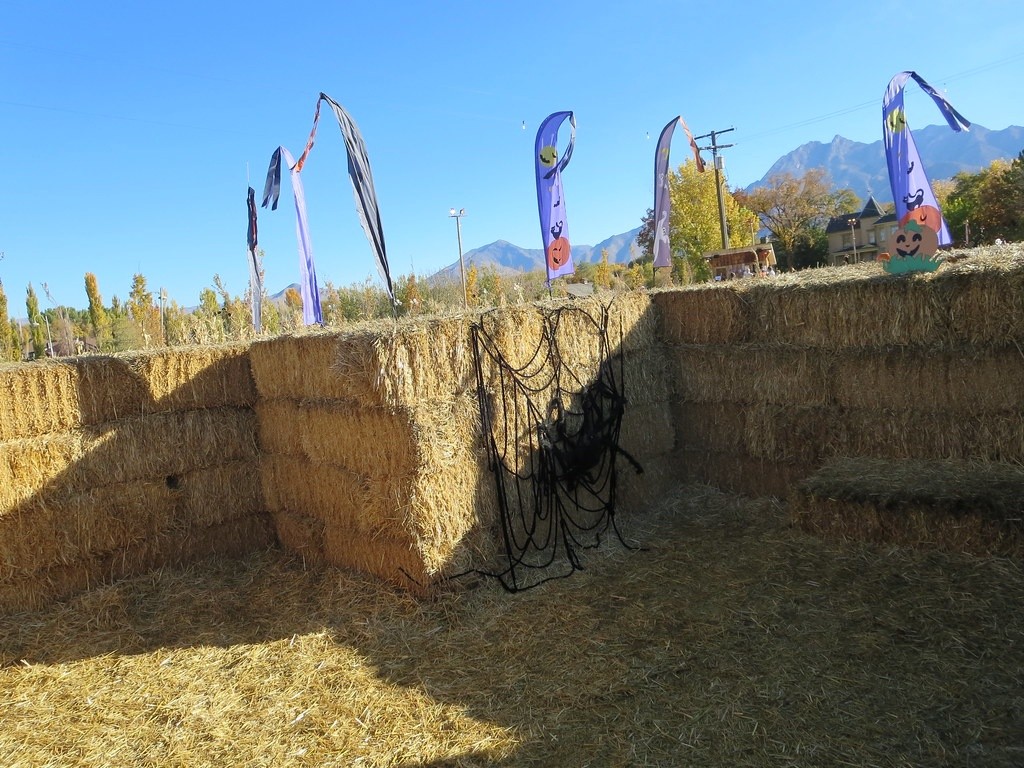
[448,207,469,311]
